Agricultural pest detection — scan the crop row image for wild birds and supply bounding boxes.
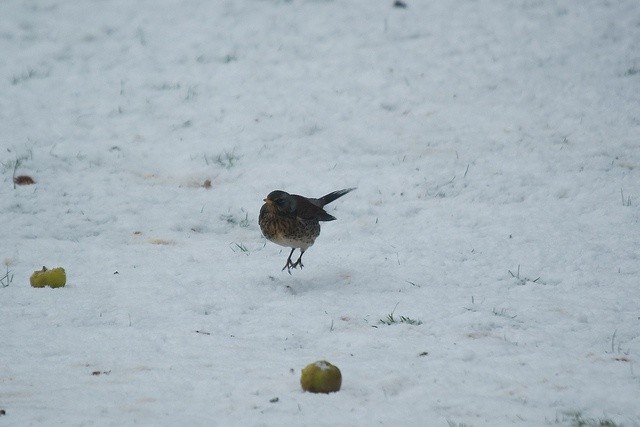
[259,187,358,276]
